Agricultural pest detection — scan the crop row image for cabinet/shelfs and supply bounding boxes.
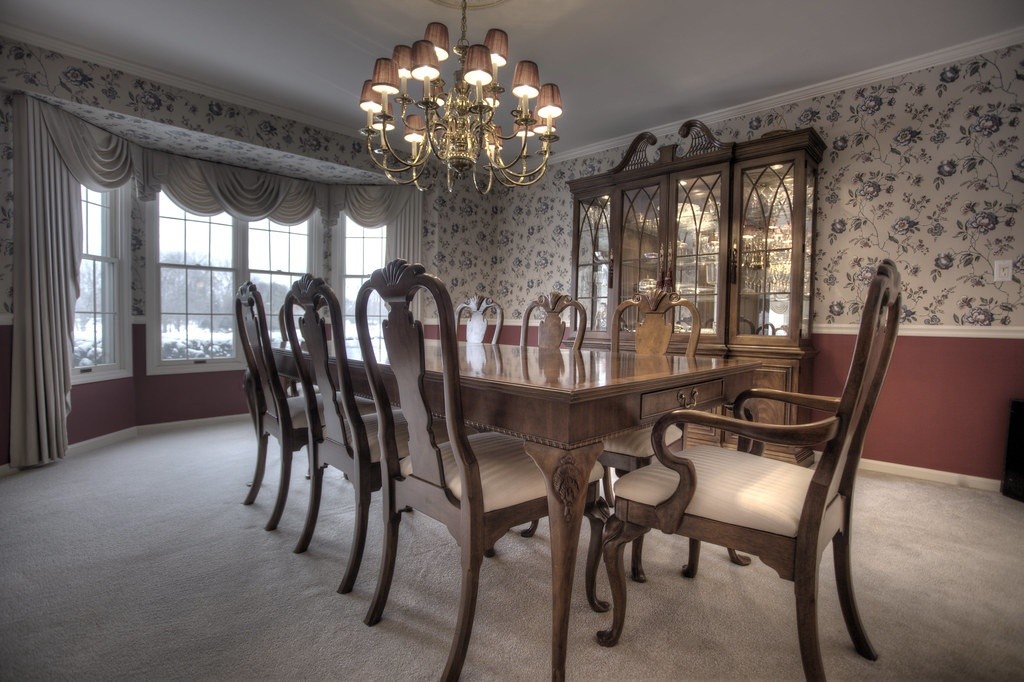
[564,119,828,467]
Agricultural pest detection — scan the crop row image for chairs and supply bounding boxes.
[227,263,923,682]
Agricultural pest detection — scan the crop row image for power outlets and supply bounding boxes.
[994,260,1013,282]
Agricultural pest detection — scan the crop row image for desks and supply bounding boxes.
[271,340,764,682]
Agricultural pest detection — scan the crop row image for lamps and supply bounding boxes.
[359,0,563,192]
